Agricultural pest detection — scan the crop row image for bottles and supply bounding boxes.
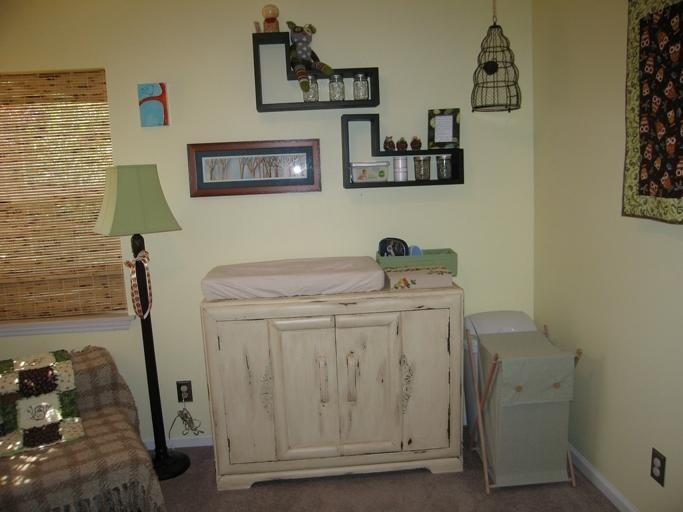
[303,74,369,102]
[392,156,455,183]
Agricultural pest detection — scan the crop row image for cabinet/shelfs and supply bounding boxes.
[200,280,464,490]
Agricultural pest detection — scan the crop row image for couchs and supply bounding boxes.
[1,344,166,510]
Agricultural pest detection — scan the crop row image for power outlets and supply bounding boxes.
[177,380,193,402]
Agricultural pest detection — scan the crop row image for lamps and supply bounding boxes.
[93,164,190,480]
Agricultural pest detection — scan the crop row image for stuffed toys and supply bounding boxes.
[286,21,334,92]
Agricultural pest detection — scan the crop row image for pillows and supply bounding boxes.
[0,350,85,456]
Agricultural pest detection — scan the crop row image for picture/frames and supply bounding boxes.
[428,108,459,149]
[187,139,321,197]
